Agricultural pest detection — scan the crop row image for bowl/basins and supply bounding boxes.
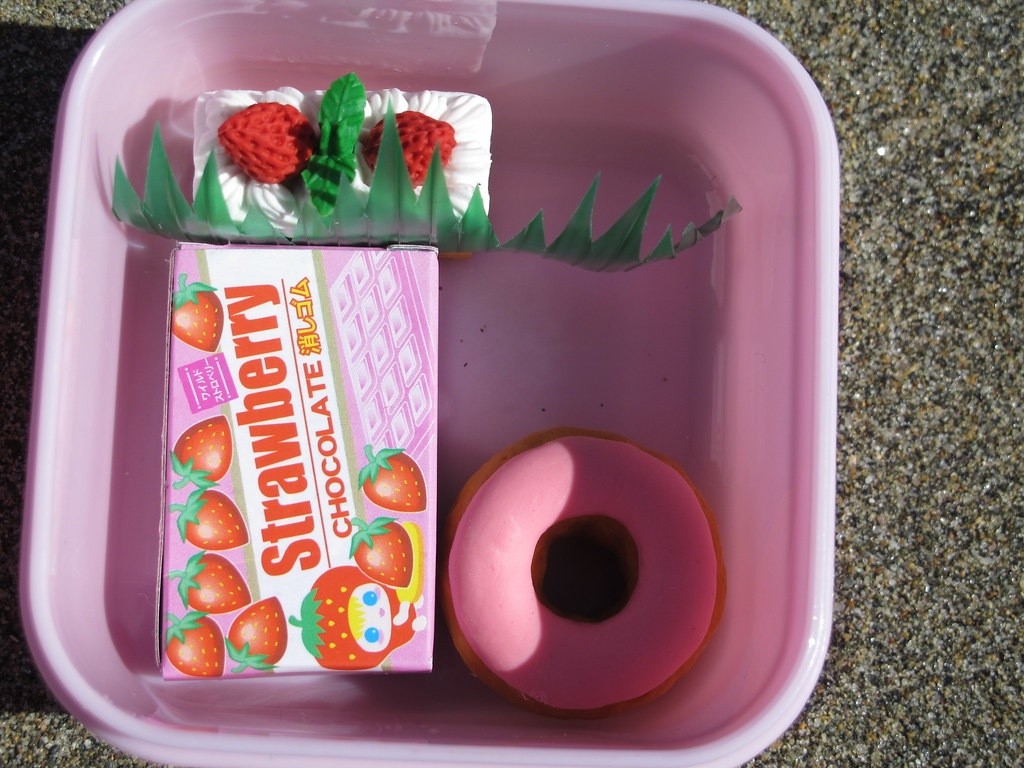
[21,0,840,768]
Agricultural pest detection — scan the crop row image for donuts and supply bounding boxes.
[440,426,728,719]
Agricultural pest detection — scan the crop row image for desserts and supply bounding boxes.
[190,73,493,228]
